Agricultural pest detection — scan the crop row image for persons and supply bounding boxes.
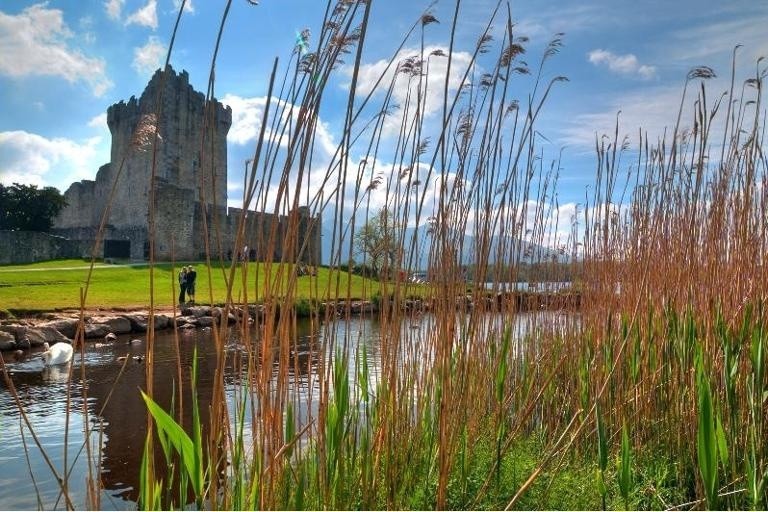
[242,244,249,262]
[178,265,197,304]
[298,264,316,277]
